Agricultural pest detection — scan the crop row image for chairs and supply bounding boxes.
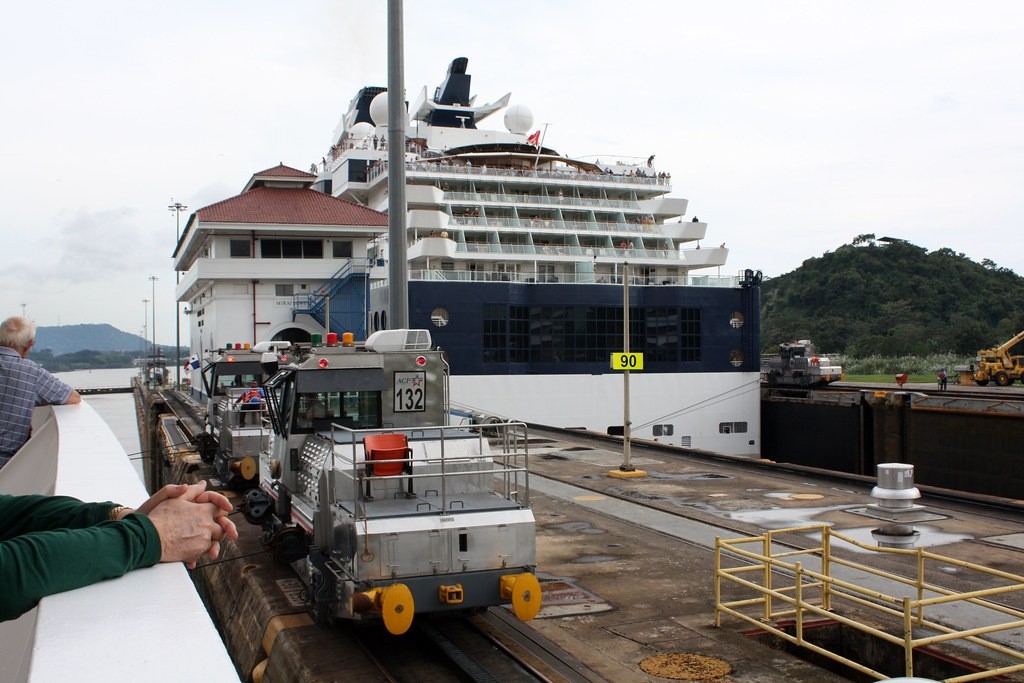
[363,434,416,499]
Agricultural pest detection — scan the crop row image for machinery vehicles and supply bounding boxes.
[972,329,1024,386]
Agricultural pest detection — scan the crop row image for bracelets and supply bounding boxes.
[109,506,133,520]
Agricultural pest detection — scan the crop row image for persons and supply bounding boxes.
[310,131,671,185]
[0,315,81,471]
[472,207,480,225]
[620,240,637,256]
[938,367,948,391]
[233,380,267,428]
[305,393,325,419]
[429,230,436,237]
[0,479,238,622]
[463,208,470,225]
[533,215,539,227]
[440,228,449,238]
[664,243,669,257]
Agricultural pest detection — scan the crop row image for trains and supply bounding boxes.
[185,328,541,636]
[760,339,844,388]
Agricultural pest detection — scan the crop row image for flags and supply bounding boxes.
[184,352,201,374]
[527,129,540,146]
